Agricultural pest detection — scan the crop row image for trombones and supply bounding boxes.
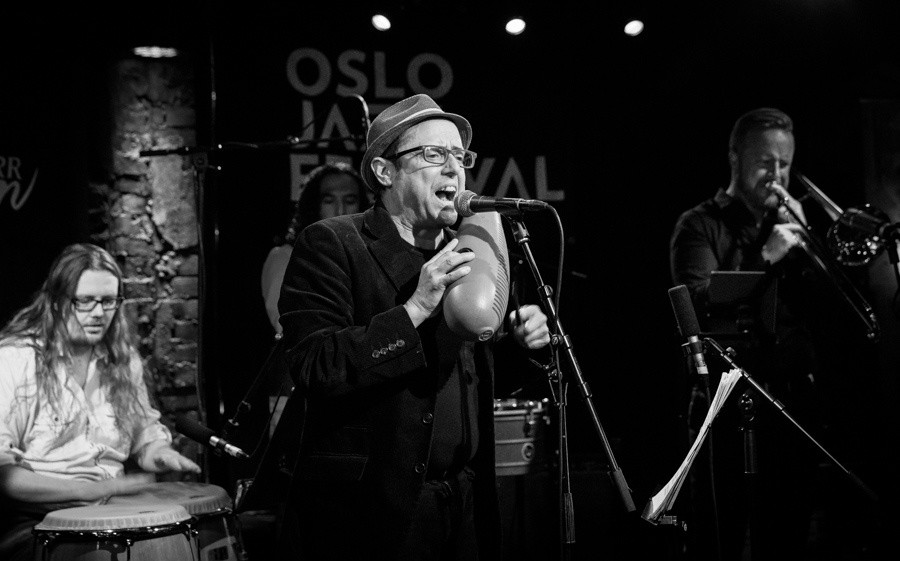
[763,171,887,339]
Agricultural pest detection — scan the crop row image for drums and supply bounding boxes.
[35,503,201,561]
[94,483,246,561]
[494,396,551,477]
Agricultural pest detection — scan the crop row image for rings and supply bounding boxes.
[784,196,789,203]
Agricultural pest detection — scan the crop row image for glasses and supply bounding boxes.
[69,297,125,312]
[385,144,477,169]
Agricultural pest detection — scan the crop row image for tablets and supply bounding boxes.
[702,271,767,336]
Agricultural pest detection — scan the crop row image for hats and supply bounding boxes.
[359,93,472,195]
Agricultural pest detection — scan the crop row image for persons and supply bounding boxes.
[258,93,557,561]
[0,240,200,561]
[672,109,878,561]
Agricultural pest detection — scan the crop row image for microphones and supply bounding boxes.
[668,284,711,387]
[841,208,900,241]
[453,189,547,218]
[173,416,251,461]
